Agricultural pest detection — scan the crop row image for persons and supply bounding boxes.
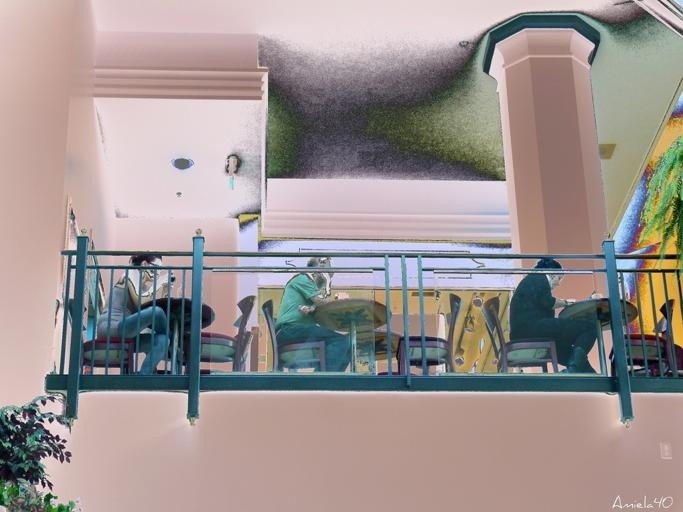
[274,256,350,374]
[95,251,185,376]
[508,257,597,376]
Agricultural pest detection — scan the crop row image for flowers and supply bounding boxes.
[0,395,81,512]
